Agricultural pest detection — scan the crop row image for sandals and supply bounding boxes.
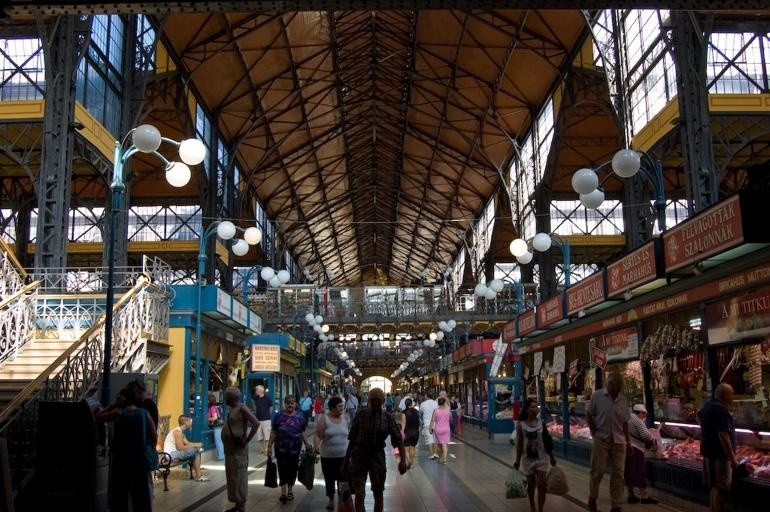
[279,493,293,503]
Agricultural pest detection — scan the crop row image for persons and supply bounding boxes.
[513,399,556,512]
[696,383,741,512]
[509,396,522,447]
[125,379,158,504]
[207,384,462,512]
[624,404,659,504]
[164,414,210,481]
[584,372,633,512]
[95,387,157,512]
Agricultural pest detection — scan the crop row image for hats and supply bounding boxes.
[633,404,648,414]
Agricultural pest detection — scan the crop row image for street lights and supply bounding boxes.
[508,228,574,288]
[571,145,671,238]
[390,318,462,398]
[102,122,208,429]
[239,262,292,401]
[300,310,363,398]
[192,220,264,433]
[470,273,525,314]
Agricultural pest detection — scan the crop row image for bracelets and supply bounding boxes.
[626,440,631,444]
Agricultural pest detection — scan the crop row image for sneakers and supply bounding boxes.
[589,495,597,511]
[640,497,658,505]
[407,459,414,469]
[428,453,447,465]
[610,506,621,512]
[628,495,640,503]
[195,475,208,482]
[225,508,245,512]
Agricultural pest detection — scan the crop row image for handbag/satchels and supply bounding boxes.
[264,460,278,487]
[137,445,157,471]
[338,481,356,512]
[298,460,314,490]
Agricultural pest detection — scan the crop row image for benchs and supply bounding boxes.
[153,452,197,491]
